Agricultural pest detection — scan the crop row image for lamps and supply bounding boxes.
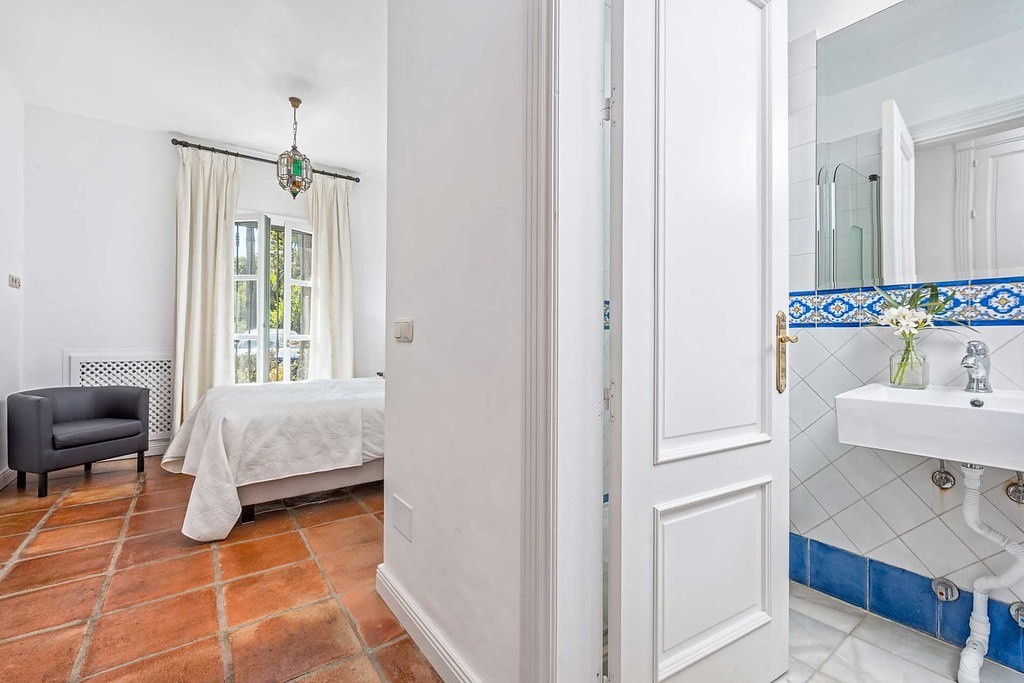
[276,97,313,199]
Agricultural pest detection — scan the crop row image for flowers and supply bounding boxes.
[873,283,980,351]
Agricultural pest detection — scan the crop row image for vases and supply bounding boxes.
[891,349,928,391]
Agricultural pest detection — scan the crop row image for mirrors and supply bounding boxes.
[816,0,1023,289]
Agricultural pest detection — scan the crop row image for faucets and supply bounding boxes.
[961,338,994,394]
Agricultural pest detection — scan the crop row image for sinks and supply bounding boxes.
[835,379,1024,474]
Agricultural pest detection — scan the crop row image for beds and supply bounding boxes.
[162,375,385,542]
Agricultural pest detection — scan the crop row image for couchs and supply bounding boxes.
[7,386,149,498]
